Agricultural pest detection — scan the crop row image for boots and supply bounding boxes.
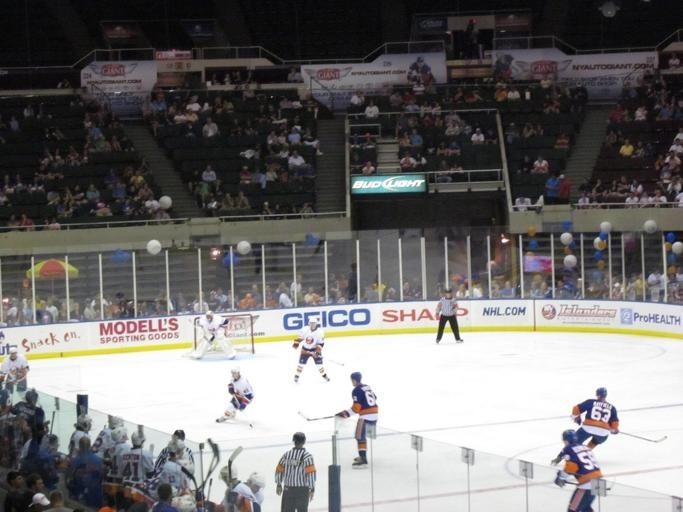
[352,455,368,465]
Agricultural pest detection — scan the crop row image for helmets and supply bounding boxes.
[595,387,608,399]
[291,431,306,443]
[25,391,38,401]
[219,465,240,481]
[563,428,579,444]
[107,415,145,447]
[75,413,94,431]
[246,472,268,490]
[165,437,185,454]
[350,371,362,383]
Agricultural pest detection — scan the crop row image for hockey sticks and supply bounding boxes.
[564,474,618,490]
[619,431,668,442]
[299,412,339,421]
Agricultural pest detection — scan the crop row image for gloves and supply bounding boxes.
[335,410,350,419]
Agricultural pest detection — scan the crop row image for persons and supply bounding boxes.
[291,317,330,383]
[189,309,237,361]
[553,428,604,512]
[0,345,269,512]
[434,288,464,343]
[336,372,379,466]
[551,386,619,465]
[1,19,683,330]
[274,430,317,512]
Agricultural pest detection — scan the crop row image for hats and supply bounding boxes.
[32,492,51,506]
